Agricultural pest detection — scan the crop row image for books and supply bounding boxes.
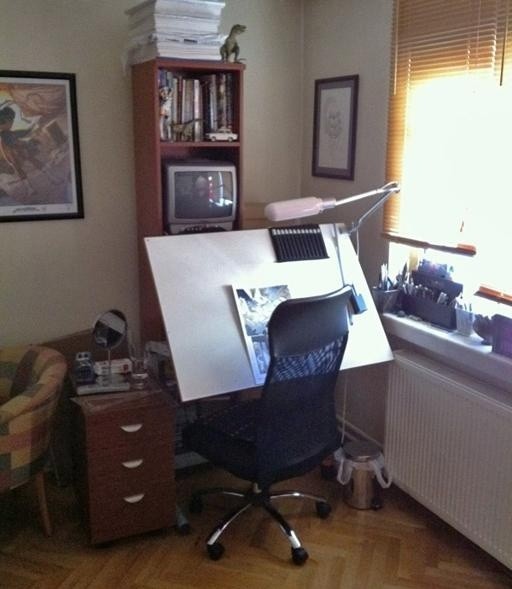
[94,359,133,375]
[158,69,234,142]
[69,370,131,396]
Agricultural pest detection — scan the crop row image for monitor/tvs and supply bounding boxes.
[162,160,238,235]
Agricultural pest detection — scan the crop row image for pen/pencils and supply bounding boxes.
[377,262,472,336]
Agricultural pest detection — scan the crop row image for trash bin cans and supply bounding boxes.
[343,442,383,510]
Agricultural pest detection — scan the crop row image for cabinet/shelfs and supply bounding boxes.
[133,57,245,425]
[68,362,180,548]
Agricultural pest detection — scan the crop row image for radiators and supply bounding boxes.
[384,349,512,571]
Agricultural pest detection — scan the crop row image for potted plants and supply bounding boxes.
[371,263,400,314]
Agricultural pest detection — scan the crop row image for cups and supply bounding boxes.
[129,348,153,390]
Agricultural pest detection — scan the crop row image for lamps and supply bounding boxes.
[265,181,400,233]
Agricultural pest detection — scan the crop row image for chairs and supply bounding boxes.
[0,343,68,538]
[183,285,352,565]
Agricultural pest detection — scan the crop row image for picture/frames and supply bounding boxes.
[312,74,359,180]
[0,70,84,221]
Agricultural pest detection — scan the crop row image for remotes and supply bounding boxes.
[76,380,131,395]
